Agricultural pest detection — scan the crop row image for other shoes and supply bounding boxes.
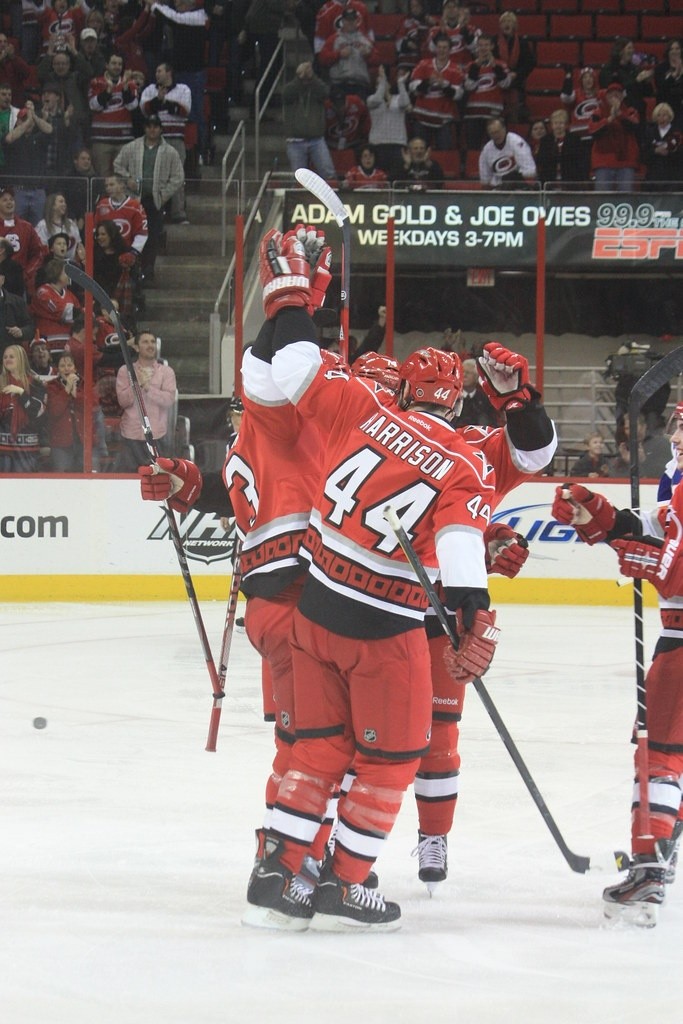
[173,217,191,225]
[143,280,155,289]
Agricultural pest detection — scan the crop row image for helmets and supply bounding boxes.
[674,394,683,419]
[320,347,464,417]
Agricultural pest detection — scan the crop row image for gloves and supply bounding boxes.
[552,482,616,546]
[609,533,683,599]
[443,608,501,686]
[138,458,202,513]
[483,522,529,580]
[261,224,333,321]
[474,342,542,413]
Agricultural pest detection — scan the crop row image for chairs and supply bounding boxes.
[0,0,256,196]
[293,0,683,192]
[94,415,139,472]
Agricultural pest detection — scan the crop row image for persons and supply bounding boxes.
[138,225,558,889]
[551,402,683,904]
[248,228,500,924]
[0,0,683,477]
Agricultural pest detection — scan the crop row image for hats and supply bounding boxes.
[341,8,359,20]
[580,67,596,80]
[0,187,15,197]
[443,0,459,7]
[28,337,48,355]
[17,109,27,119]
[605,83,623,94]
[614,38,630,52]
[329,89,346,109]
[41,82,59,93]
[143,114,161,126]
[80,28,98,40]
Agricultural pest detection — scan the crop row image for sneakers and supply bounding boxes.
[412,830,449,882]
[248,826,401,924]
[603,822,683,905]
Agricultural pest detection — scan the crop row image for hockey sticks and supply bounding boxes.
[629,345,683,835]
[64,263,225,699]
[294,167,352,365]
[382,504,631,875]
[205,538,244,752]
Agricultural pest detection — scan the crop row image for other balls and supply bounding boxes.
[33,717,47,729]
